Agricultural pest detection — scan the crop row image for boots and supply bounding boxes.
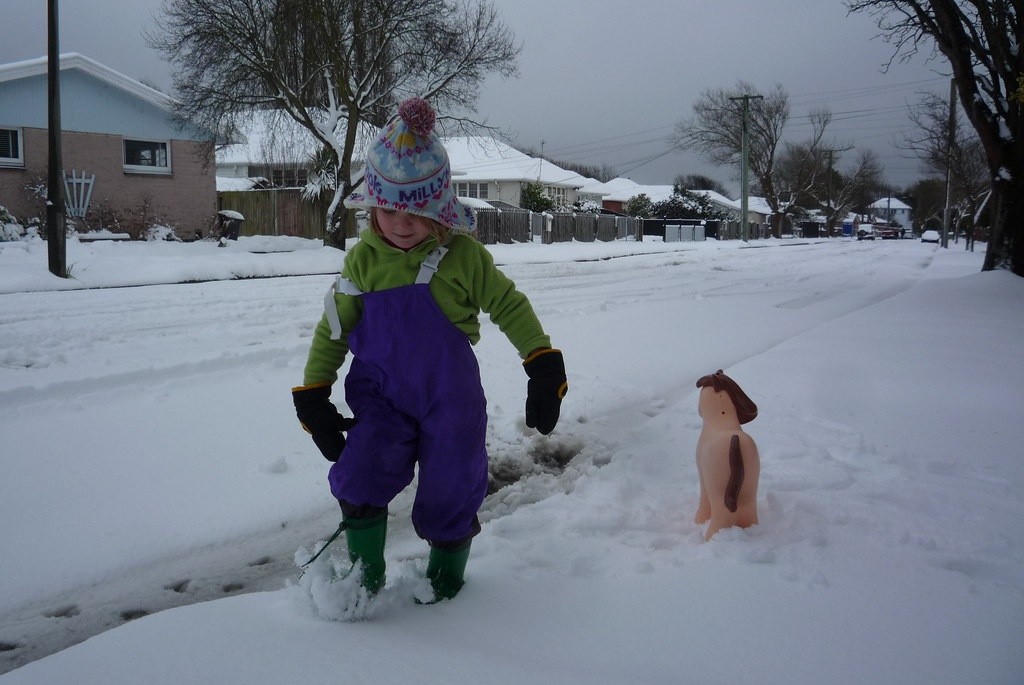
[340,512,388,609]
[413,544,471,606]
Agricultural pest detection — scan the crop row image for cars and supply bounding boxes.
[920,230,941,243]
[882,229,896,239]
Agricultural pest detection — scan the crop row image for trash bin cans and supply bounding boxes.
[217,212,244,242]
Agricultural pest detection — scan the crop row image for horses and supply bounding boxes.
[696,369,760,540]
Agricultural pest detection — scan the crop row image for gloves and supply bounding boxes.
[291,384,356,464]
[522,348,568,437]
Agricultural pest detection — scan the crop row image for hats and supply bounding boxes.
[343,99,478,234]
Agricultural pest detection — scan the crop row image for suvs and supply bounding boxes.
[857,224,875,240]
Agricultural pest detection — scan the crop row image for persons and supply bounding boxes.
[290,97,568,603]
[901,228,905,238]
